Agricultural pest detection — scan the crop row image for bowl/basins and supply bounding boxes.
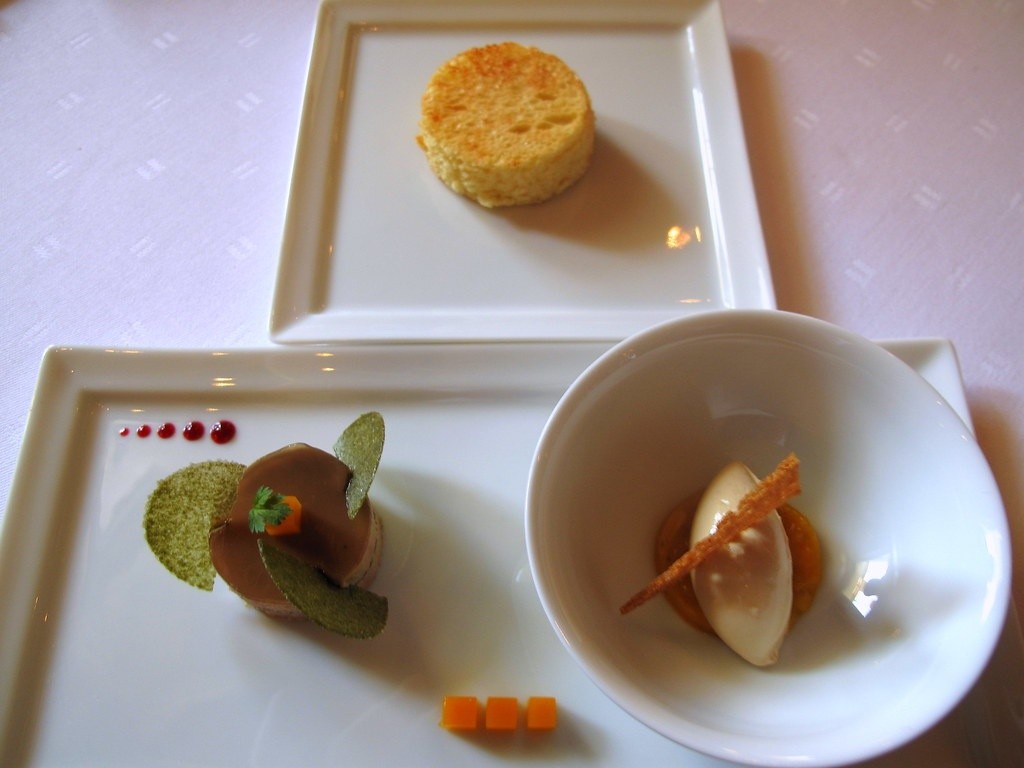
[524,309,1012,768]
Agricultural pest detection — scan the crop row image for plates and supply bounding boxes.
[1,337,1024,768]
[263,0,778,345]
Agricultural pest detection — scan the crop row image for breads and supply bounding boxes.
[417,42,595,207]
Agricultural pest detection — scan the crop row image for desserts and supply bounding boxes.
[142,410,389,640]
[620,452,822,666]
